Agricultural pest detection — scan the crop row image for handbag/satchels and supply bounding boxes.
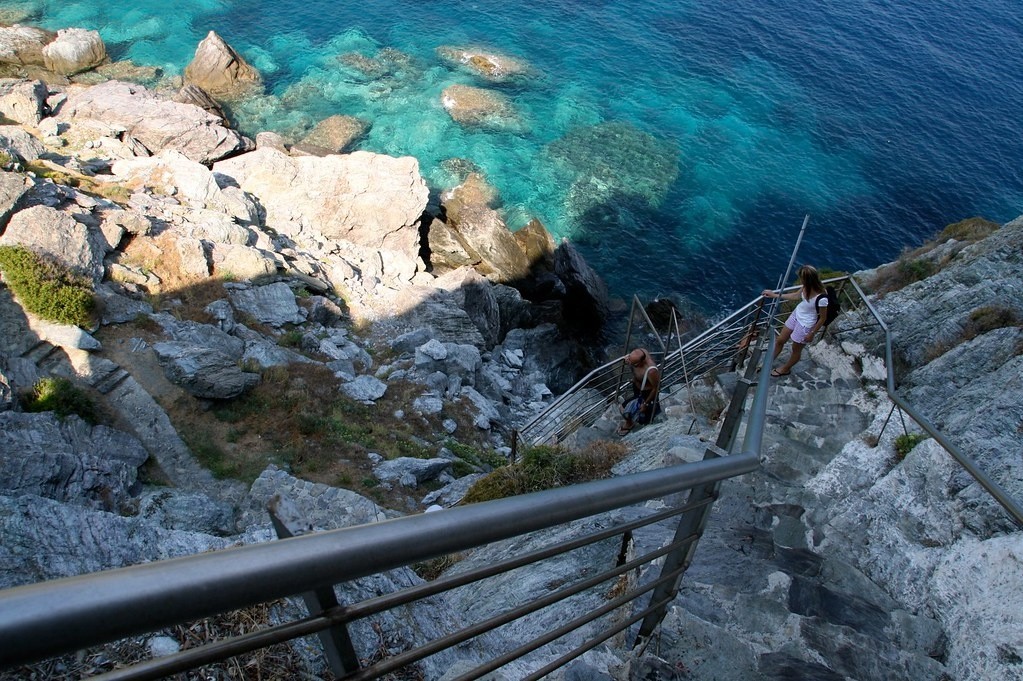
[638,402,662,426]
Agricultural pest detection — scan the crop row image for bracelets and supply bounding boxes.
[809,329,815,334]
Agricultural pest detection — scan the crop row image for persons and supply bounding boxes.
[616,348,660,437]
[756,265,828,377]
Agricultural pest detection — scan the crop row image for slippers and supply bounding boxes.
[618,422,635,437]
[756,365,774,372]
[771,368,791,376]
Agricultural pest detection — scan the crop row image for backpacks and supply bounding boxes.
[815,286,840,325]
